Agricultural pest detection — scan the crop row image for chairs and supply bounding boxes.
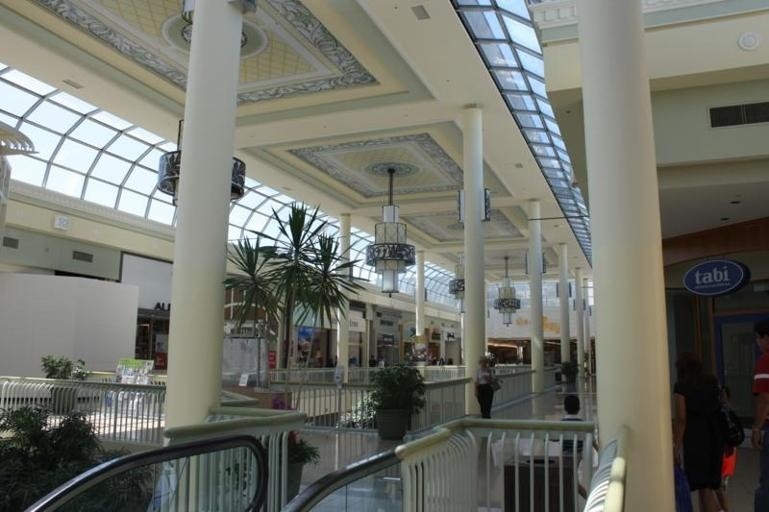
[543,439,598,465]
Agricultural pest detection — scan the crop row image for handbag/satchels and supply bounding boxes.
[489,382,500,391]
[720,403,744,446]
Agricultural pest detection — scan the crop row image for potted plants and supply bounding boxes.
[355,360,425,440]
[41,353,89,414]
[220,200,368,507]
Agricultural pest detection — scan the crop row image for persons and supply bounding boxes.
[550,394,598,467]
[749,320,769,512]
[667,333,734,512]
[473,354,497,418]
[280,340,453,368]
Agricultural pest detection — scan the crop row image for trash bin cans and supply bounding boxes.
[505,439,583,512]
[555,372,561,381]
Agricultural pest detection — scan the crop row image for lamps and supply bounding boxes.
[181,0,197,24]
[158,116,246,238]
[447,251,466,313]
[366,167,416,297]
[493,256,521,326]
[457,188,491,223]
